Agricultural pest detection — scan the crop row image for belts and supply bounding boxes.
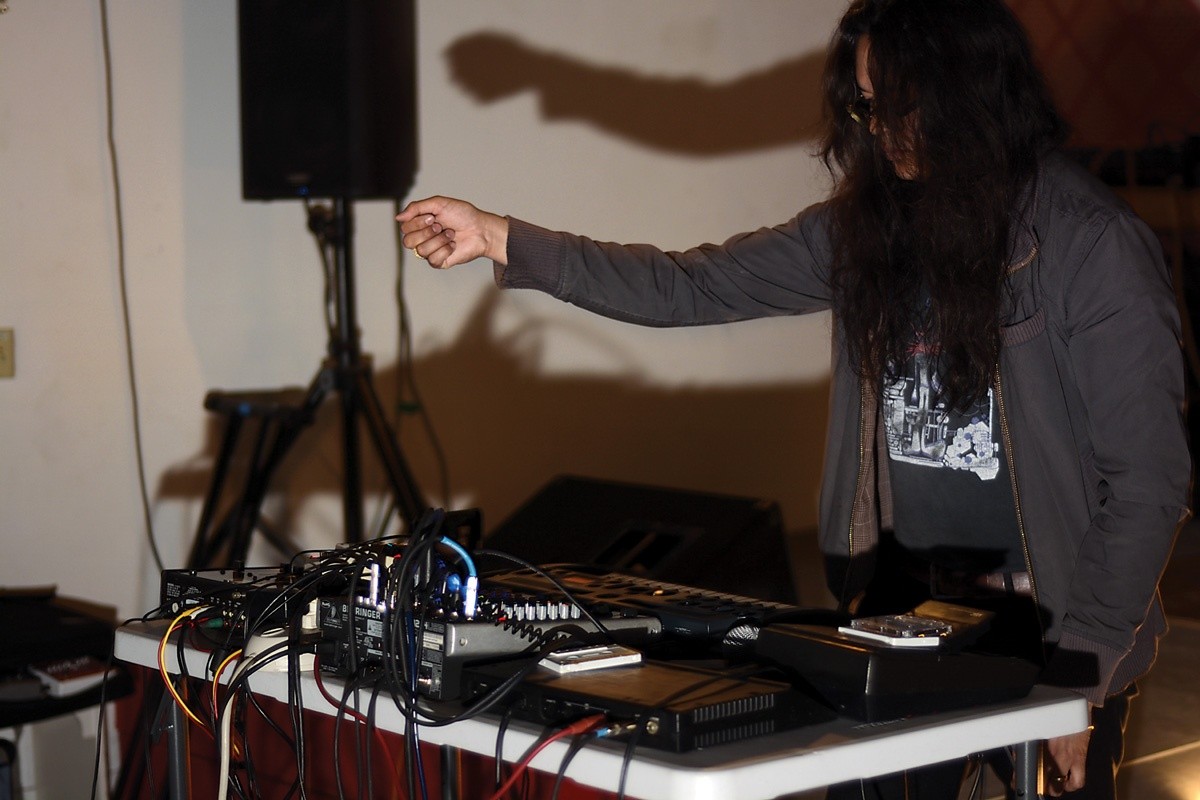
[896,560,1035,600]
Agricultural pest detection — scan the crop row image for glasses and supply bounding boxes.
[845,95,920,126]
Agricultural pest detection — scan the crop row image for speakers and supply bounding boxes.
[235,0,419,201]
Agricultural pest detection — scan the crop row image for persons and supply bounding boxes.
[393,0,1196,800]
